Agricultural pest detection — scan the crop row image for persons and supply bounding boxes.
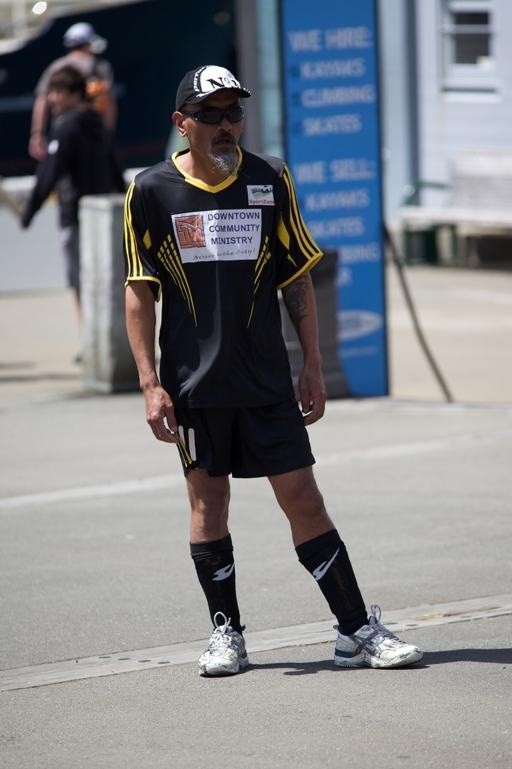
[27,21,118,161]
[121,63,425,677]
[16,64,127,365]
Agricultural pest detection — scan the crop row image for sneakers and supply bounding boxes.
[332,617,423,669]
[199,625,249,677]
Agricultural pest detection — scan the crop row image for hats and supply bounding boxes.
[64,22,107,53]
[48,65,95,102]
[176,65,252,113]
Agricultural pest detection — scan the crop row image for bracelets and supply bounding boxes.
[29,128,46,140]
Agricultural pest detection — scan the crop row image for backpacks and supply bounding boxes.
[85,59,116,130]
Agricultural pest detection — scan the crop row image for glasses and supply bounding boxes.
[182,105,244,126]
